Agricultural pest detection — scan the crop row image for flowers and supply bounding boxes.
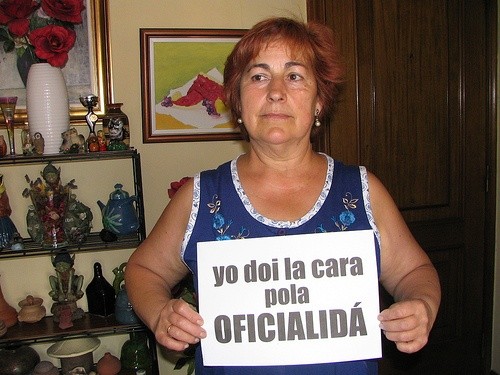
[0,0,86,82]
[167,176,190,201]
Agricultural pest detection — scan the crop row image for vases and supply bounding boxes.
[102,103,130,147]
[27,62,71,155]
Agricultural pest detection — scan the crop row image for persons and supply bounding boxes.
[97,130,107,151]
[125,4,442,375]
[49,251,84,302]
[86,131,100,152]
[36,164,69,244]
[0,174,24,250]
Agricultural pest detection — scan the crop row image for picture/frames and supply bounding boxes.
[0,0,115,128]
[140,28,250,144]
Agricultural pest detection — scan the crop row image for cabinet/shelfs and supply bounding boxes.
[0,149,161,375]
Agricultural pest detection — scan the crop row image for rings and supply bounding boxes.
[167,325,173,333]
